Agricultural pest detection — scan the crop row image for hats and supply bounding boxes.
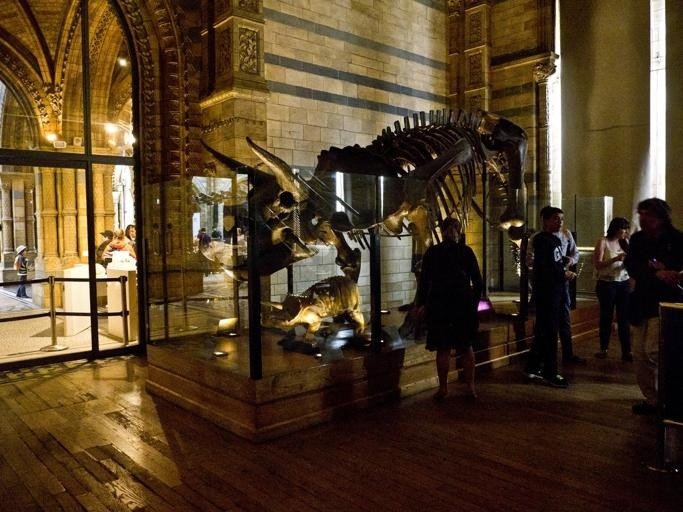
[16,245,26,253]
[100,230,113,238]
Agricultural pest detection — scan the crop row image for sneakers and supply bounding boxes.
[522,351,658,414]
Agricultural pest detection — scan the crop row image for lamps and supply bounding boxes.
[103,120,136,149]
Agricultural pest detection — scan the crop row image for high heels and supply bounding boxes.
[433,391,448,399]
[465,390,476,399]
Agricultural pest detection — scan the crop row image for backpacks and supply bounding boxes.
[14,257,20,271]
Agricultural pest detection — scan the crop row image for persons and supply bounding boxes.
[592,217,636,361]
[522,205,580,386]
[14,245,30,298]
[413,218,483,398]
[624,197,683,415]
[197,226,222,246]
[96,225,137,266]
